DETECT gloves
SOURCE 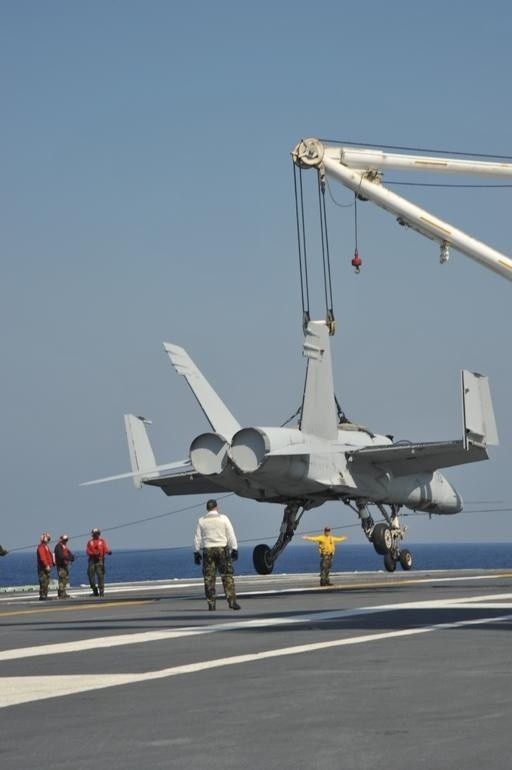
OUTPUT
[231,549,238,562]
[194,551,202,565]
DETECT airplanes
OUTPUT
[75,318,499,575]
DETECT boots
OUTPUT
[100,589,104,597]
[57,590,70,599]
[89,590,98,597]
[40,593,52,601]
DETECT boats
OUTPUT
[0,138,511,770]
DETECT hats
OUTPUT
[206,499,217,508]
[324,527,331,530]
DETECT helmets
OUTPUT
[59,534,69,543]
[90,528,101,538]
[41,532,51,543]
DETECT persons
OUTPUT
[54,534,74,599]
[193,500,241,610]
[303,527,347,587]
[37,533,54,599]
[86,528,112,597]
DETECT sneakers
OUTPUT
[208,605,215,611]
[229,599,241,610]
[319,581,334,586]
